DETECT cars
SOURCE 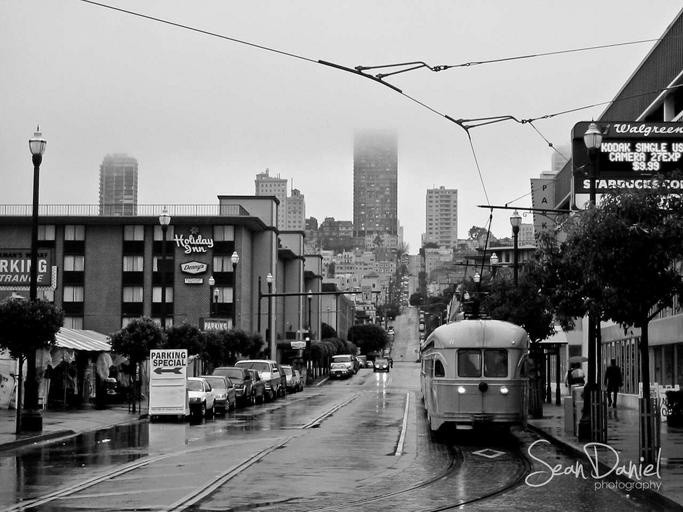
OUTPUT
[419,310,425,343]
[387,325,397,335]
[187,359,304,416]
[329,347,393,379]
[402,275,409,308]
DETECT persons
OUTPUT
[389,357,393,369]
[603,357,624,409]
[564,362,584,397]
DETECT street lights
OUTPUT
[307,290,313,337]
[578,119,602,443]
[266,272,273,358]
[159,204,171,330]
[208,276,220,318]
[462,251,499,315]
[20,122,48,432]
[510,208,522,280]
[231,250,239,330]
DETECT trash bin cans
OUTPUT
[665,390,683,428]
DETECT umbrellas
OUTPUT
[566,355,588,364]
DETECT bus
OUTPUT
[420,320,530,444]
